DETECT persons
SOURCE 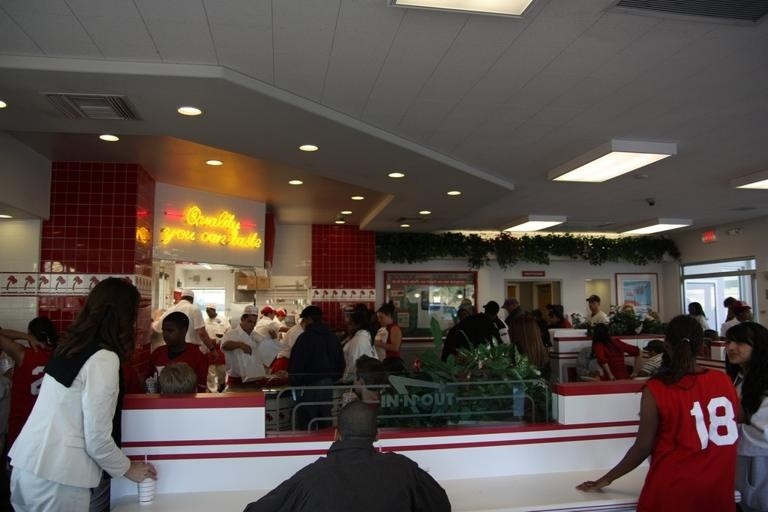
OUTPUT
[0,315,60,454]
[722,320,768,512]
[440,294,752,382]
[575,316,747,511]
[4,278,158,512]
[143,287,407,432]
[243,401,453,512]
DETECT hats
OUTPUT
[300,306,323,318]
[586,295,601,302]
[242,304,258,316]
[205,303,216,310]
[260,305,287,317]
[181,290,194,299]
[502,298,519,309]
[642,340,665,353]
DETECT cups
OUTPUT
[137,465,156,506]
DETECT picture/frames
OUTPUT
[615,273,660,314]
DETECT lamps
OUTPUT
[614,217,694,237]
[388,0,533,21]
[546,140,678,185]
[730,169,767,192]
[497,213,566,233]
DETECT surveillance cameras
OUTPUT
[648,199,655,205]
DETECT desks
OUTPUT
[111,463,652,511]
